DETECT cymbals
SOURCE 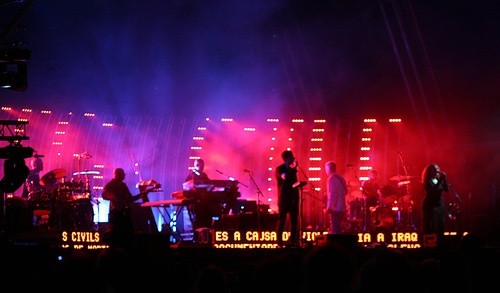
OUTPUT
[41,168,66,184]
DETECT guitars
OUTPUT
[110,183,161,210]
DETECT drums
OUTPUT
[378,185,396,205]
[348,201,364,222]
[370,204,397,231]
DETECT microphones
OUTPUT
[215,169,223,174]
[437,170,444,174]
[244,169,249,172]
[346,164,352,166]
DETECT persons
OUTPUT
[422,163,448,233]
[276,151,300,247]
[185,159,209,185]
[361,170,378,230]
[0,224,500,293]
[326,161,345,233]
[102,168,143,222]
[42,173,57,189]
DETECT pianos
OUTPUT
[140,198,192,242]
